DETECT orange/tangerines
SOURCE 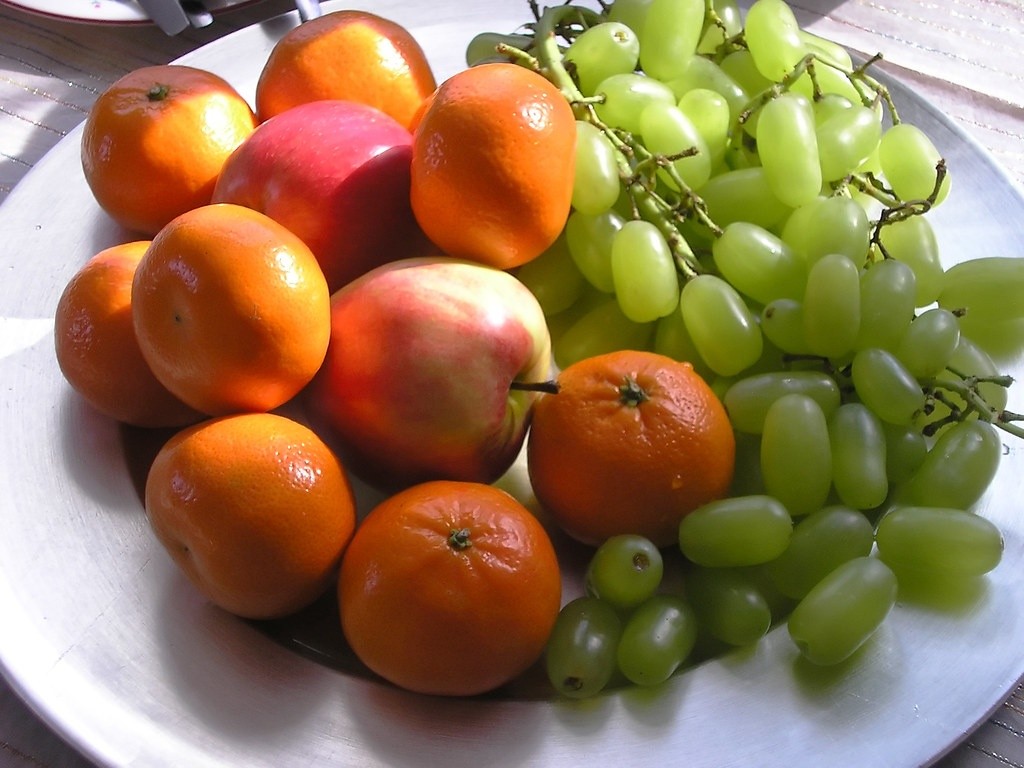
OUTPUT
[56,10,734,697]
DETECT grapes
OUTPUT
[466,0,1024,692]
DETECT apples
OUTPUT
[304,257,551,481]
[211,100,413,285]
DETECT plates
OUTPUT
[0,0,275,26]
[0,0,1024,768]
